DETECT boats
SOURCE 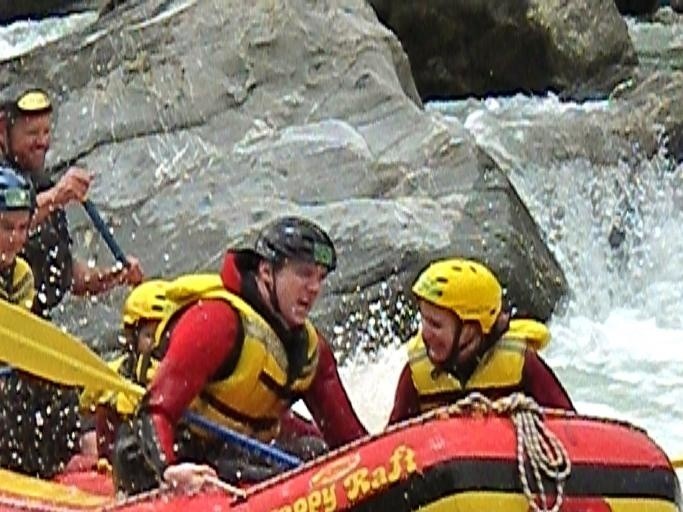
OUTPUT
[0,403,683,511]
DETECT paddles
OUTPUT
[0,300,305,467]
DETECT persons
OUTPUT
[380,256,578,419]
[0,164,83,480]
[0,79,146,329]
[86,276,178,471]
[114,212,372,490]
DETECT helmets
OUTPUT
[122,277,172,326]
[411,255,504,335]
[254,215,337,272]
[0,82,54,116]
[0,163,35,213]
